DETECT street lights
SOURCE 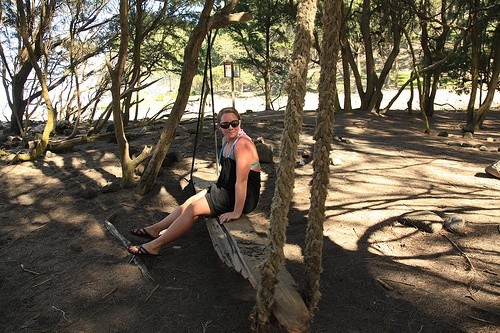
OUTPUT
[224,61,240,109]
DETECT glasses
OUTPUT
[219,119,241,129]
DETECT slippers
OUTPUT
[126,245,159,257]
[127,227,160,239]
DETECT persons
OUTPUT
[127,107,261,257]
